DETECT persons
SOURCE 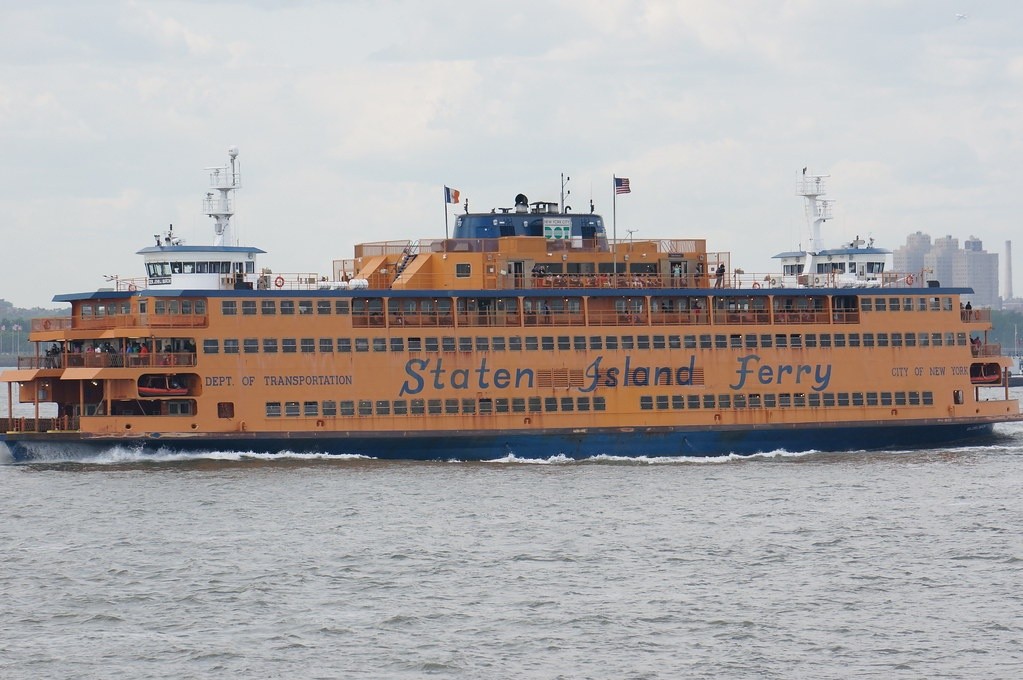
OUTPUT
[371,312,379,323]
[625,300,700,321]
[671,264,699,287]
[714,264,725,289]
[732,304,815,322]
[544,300,550,324]
[237,269,243,282]
[157,343,196,365]
[120,342,149,366]
[46,343,81,368]
[397,314,402,323]
[342,272,352,281]
[961,301,982,355]
[532,265,664,288]
[86,342,117,367]
[258,274,265,289]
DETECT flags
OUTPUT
[445,187,460,204]
[615,178,631,195]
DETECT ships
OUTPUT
[1,143,1022,460]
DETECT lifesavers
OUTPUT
[166,355,177,366]
[274,276,285,288]
[128,283,138,292]
[905,274,914,285]
[752,283,760,289]
[770,279,776,285]
[974,310,981,320]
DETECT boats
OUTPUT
[137,386,189,397]
[970,365,999,384]
